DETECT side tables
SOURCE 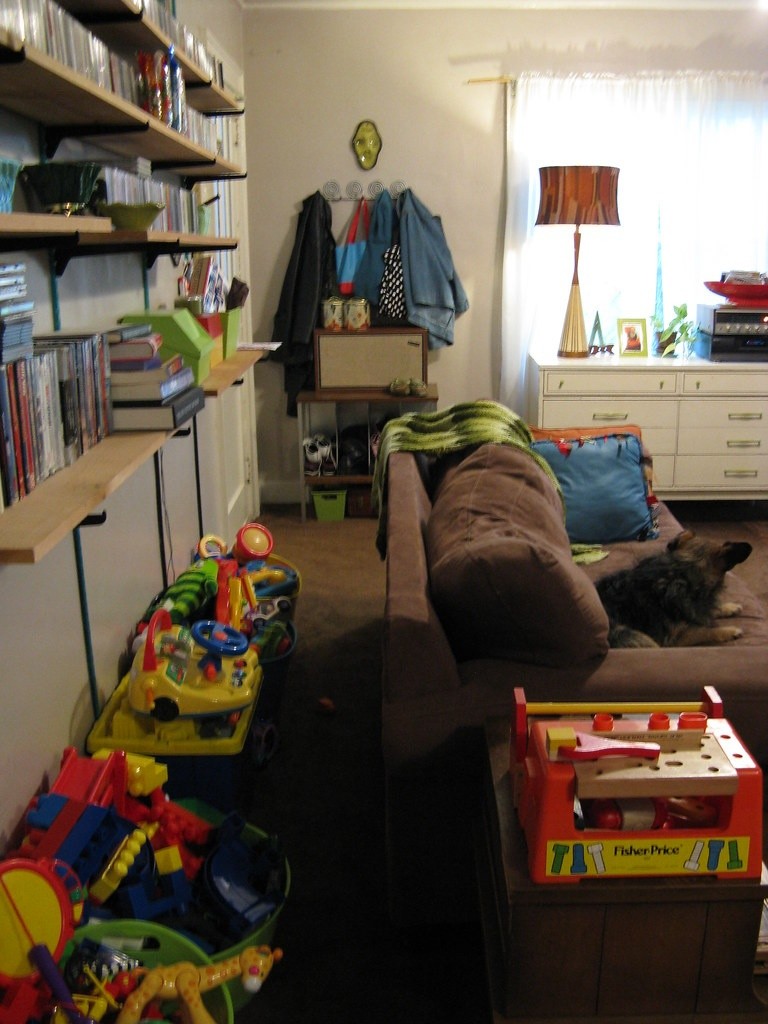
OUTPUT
[478,713,768,1024]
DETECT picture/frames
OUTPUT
[617,317,648,357]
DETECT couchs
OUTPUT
[372,398,768,926]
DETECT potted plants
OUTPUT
[650,304,696,359]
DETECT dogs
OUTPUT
[590,528,754,648]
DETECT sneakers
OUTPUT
[302,430,337,477]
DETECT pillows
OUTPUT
[426,443,609,667]
[533,432,655,544]
[528,425,642,443]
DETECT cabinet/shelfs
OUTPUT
[297,383,439,520]
[311,326,428,392]
[527,353,768,501]
[0,0,264,567]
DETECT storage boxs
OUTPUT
[257,551,302,620]
[59,918,235,1024]
[207,816,291,1008]
[309,486,348,522]
[218,306,242,360]
[257,613,297,683]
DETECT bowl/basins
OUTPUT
[703,281,768,308]
[17,160,167,233]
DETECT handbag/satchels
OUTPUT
[336,196,372,297]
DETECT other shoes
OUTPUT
[339,426,368,475]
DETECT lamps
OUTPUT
[535,166,620,359]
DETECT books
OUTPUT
[0,1,223,234]
[0,262,204,507]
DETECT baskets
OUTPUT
[348,491,377,518]
